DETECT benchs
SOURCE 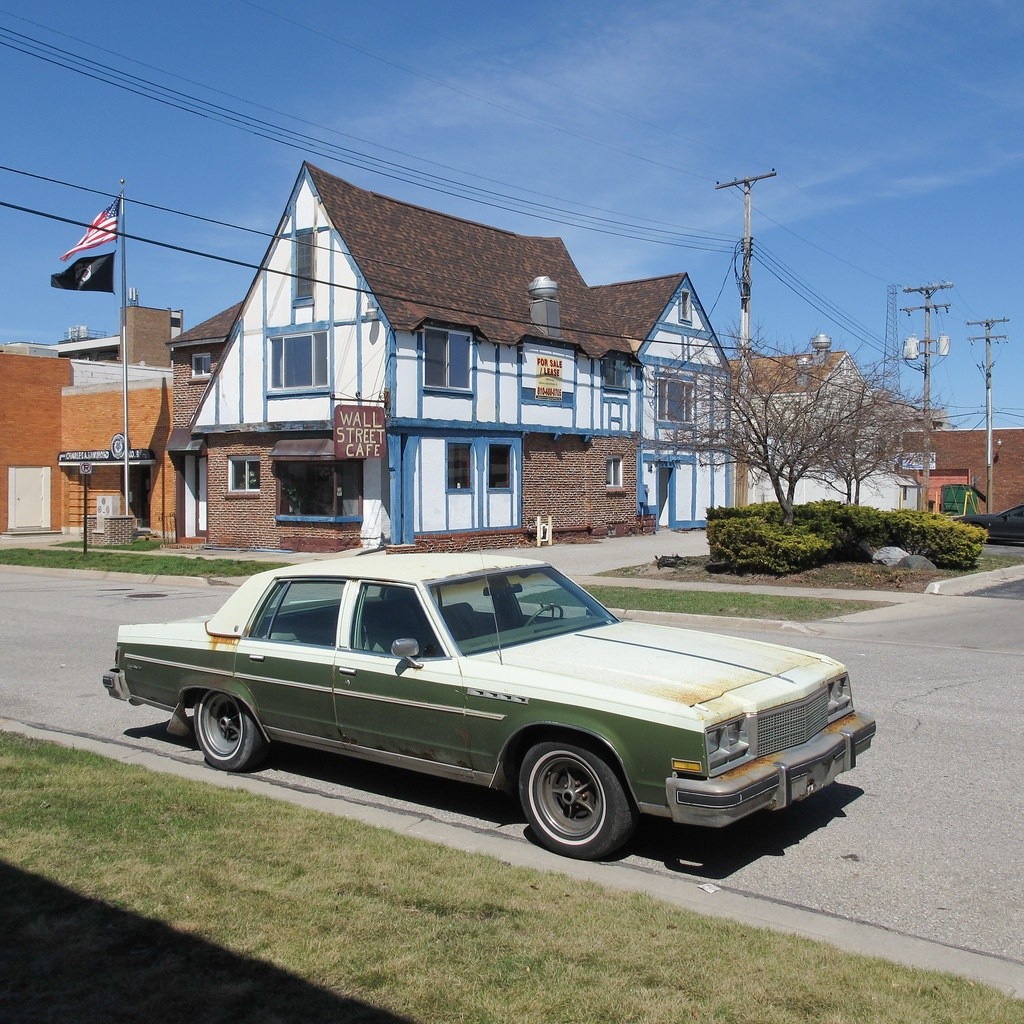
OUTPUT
[259,598,389,645]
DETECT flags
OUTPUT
[59,193,120,261]
[50,252,113,293]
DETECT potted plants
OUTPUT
[288,488,302,516]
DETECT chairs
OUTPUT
[366,602,431,656]
[437,600,480,641]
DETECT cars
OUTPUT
[953,502,1024,544]
[102,552,875,860]
